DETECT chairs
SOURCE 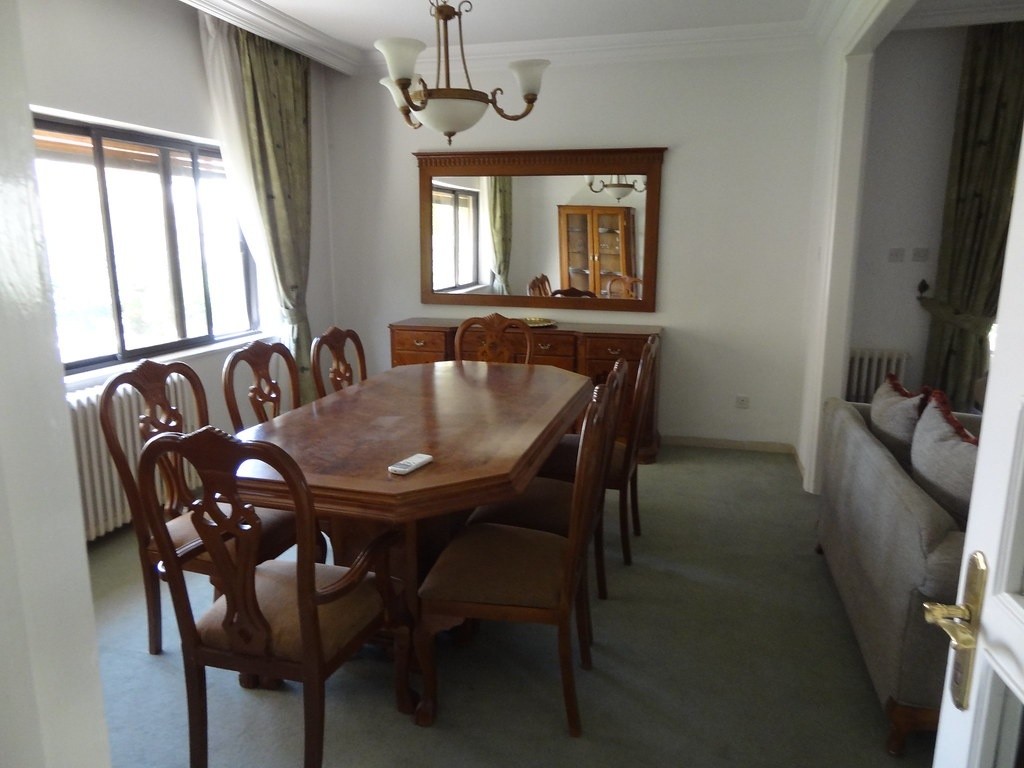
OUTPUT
[527,273,552,297]
[98,313,663,767]
[550,288,597,300]
[607,276,644,300]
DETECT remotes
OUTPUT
[389,453,433,475]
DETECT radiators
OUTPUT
[847,349,906,405]
[66,373,203,541]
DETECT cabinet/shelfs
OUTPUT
[387,317,664,464]
[556,204,638,299]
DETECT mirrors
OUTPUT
[413,147,669,313]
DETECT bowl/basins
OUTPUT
[599,227,609,233]
[569,267,583,273]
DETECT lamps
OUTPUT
[583,175,646,203]
[374,0,551,146]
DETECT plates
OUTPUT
[614,230,619,233]
[510,317,559,327]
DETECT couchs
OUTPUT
[813,397,982,754]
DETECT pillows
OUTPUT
[911,391,979,532]
[869,373,932,475]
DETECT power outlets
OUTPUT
[735,393,749,410]
[888,248,906,262]
[911,248,928,262]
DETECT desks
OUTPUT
[211,360,594,712]
